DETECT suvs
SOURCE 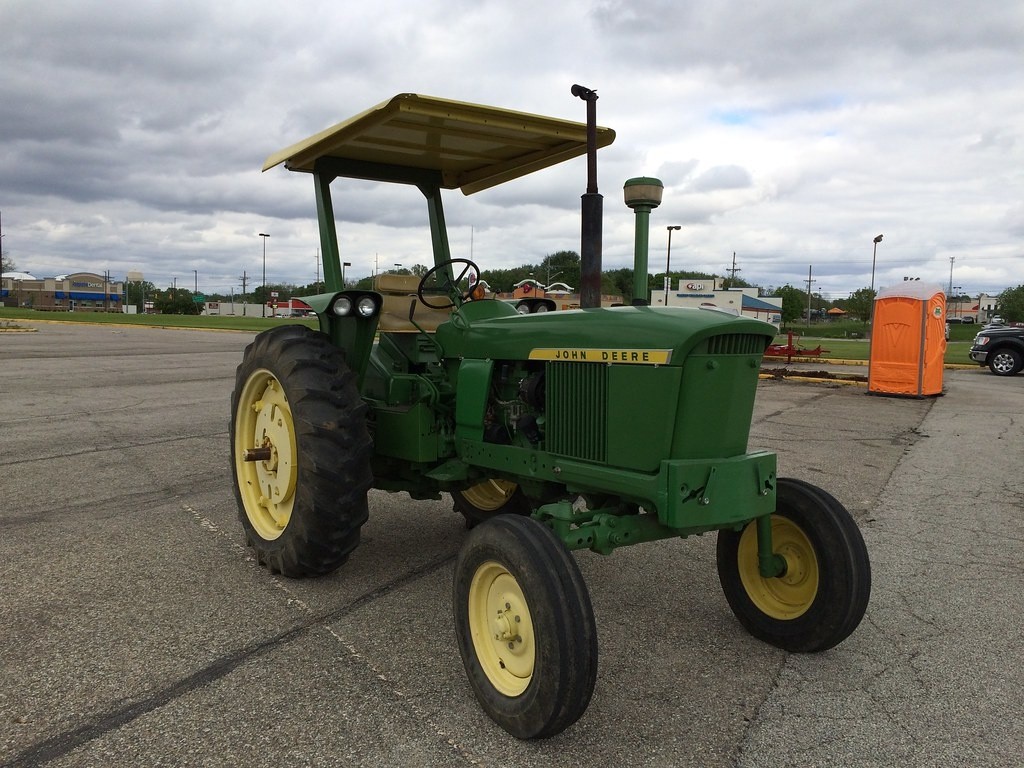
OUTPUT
[961,315,974,324]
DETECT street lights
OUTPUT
[953,287,961,317]
[959,293,966,316]
[395,264,402,274]
[665,226,681,305]
[871,234,883,324]
[342,262,351,286]
[547,271,563,286]
[258,233,270,318]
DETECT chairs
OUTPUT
[376,274,453,334]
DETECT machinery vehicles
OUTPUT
[228,83,871,741]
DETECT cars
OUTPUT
[982,323,1005,329]
[275,313,301,319]
[969,327,1024,375]
[992,316,1004,324]
